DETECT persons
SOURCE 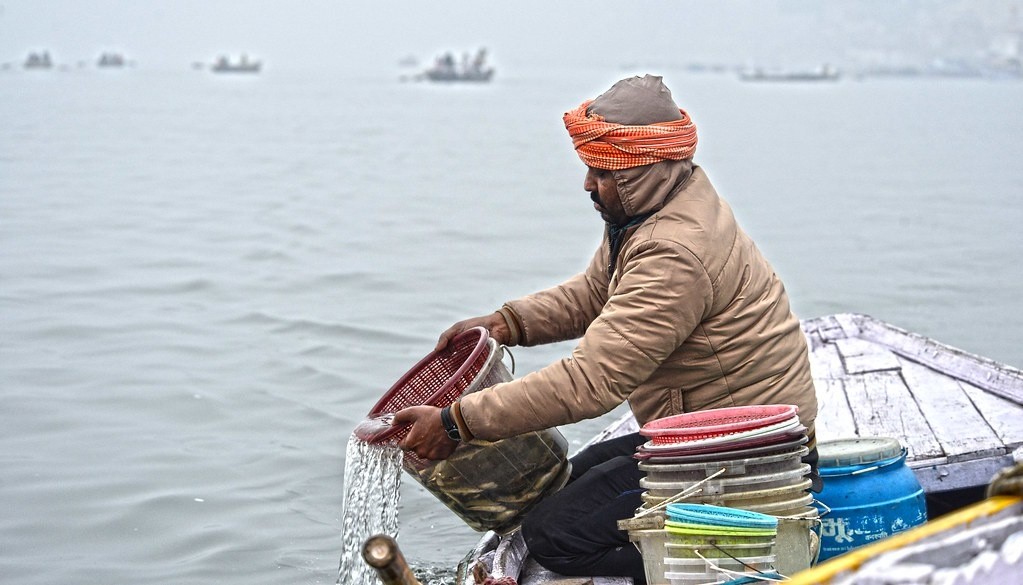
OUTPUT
[391,75,823,577]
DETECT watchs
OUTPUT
[440,406,462,442]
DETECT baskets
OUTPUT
[633,403,831,585]
[357,325,504,471]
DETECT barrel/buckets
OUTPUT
[354,336,573,537]
[812,434,927,563]
[616,404,830,584]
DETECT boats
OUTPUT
[427,69,494,81]
[740,71,842,79]
[212,60,261,72]
